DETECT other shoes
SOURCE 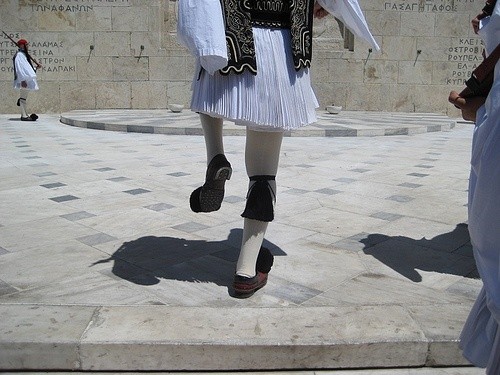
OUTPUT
[190,154,232,212]
[21,114,38,121]
[233,247,272,298]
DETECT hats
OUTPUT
[18,40,27,45]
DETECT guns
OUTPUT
[2,30,42,68]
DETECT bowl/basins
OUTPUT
[169,104,184,112]
[326,106,342,114]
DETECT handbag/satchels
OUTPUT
[455,44,500,121]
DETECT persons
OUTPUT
[12,39,41,121]
[449,1,500,375]
[178,0,379,298]
